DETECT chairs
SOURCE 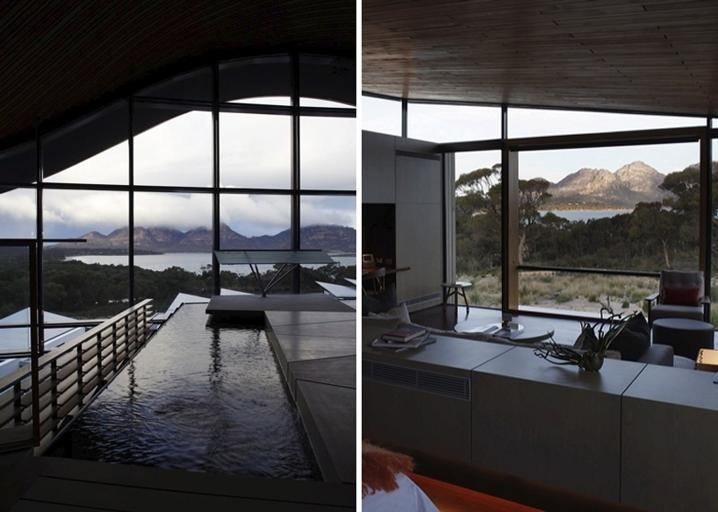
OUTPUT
[646,270,712,327]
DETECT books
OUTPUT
[380,322,427,342]
[374,336,438,354]
[370,332,430,350]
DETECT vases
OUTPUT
[584,351,604,372]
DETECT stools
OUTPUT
[652,318,715,361]
[440,281,472,314]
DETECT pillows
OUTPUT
[366,301,412,323]
